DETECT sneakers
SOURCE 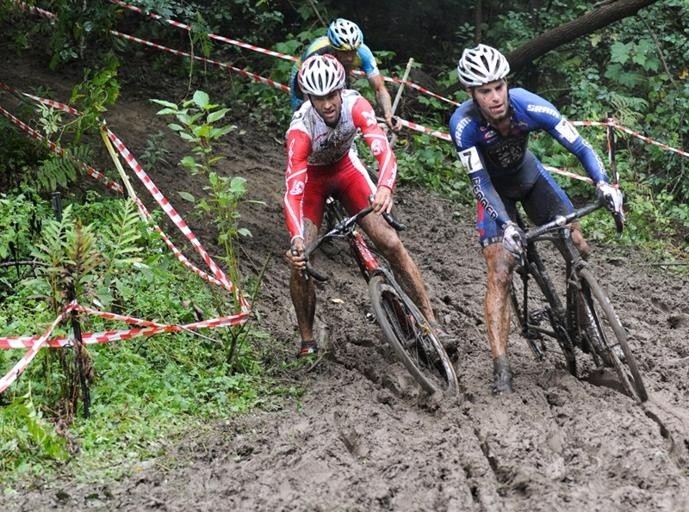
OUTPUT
[432,325,459,357]
[298,338,318,365]
[595,344,627,365]
[492,367,514,400]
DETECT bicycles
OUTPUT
[291,193,460,402]
[502,185,648,403]
[354,115,402,240]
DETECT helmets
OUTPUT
[457,43,511,89]
[297,52,346,98]
[327,18,364,51]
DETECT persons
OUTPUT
[449,41,629,407]
[280,55,463,374]
[286,16,405,138]
